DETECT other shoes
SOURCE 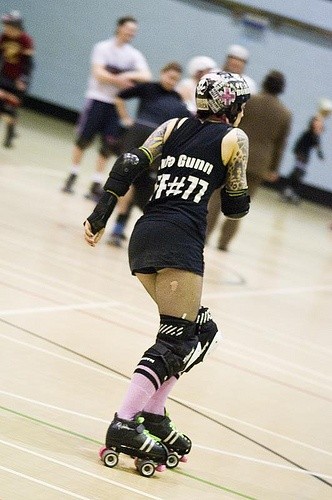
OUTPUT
[4,139,14,147]
[89,183,103,197]
[63,184,73,194]
[85,192,104,202]
[109,232,128,247]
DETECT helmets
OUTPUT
[189,55,216,77]
[194,70,252,122]
[1,8,23,26]
[227,44,250,63]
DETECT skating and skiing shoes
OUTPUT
[99,411,169,476]
[135,409,192,468]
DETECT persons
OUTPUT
[0,9,35,150]
[60,16,332,252]
[82,70,252,476]
